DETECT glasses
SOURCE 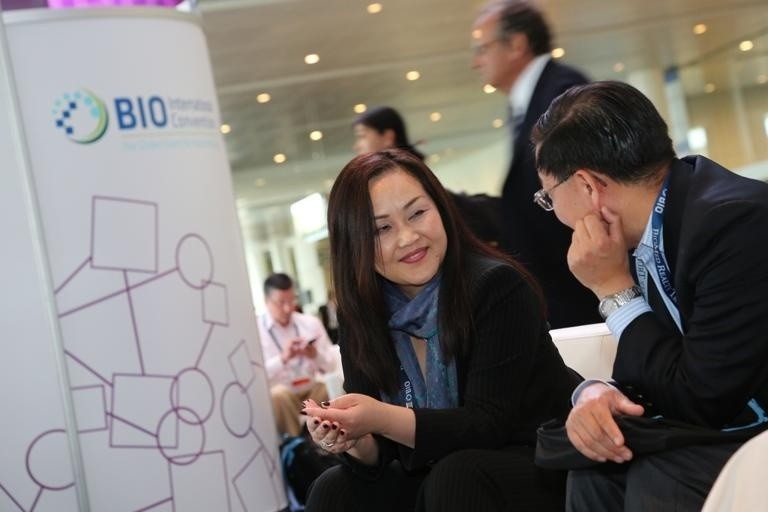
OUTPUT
[534,169,607,213]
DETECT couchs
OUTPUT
[539,319,767,510]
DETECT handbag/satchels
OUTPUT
[530,411,700,468]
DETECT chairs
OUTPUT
[314,343,348,403]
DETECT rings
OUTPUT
[322,441,333,447]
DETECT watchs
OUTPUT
[598,286,644,320]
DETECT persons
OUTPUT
[350,106,426,161]
[527,81,768,511]
[258,272,337,440]
[299,148,585,512]
[470,0,604,329]
[318,289,341,344]
